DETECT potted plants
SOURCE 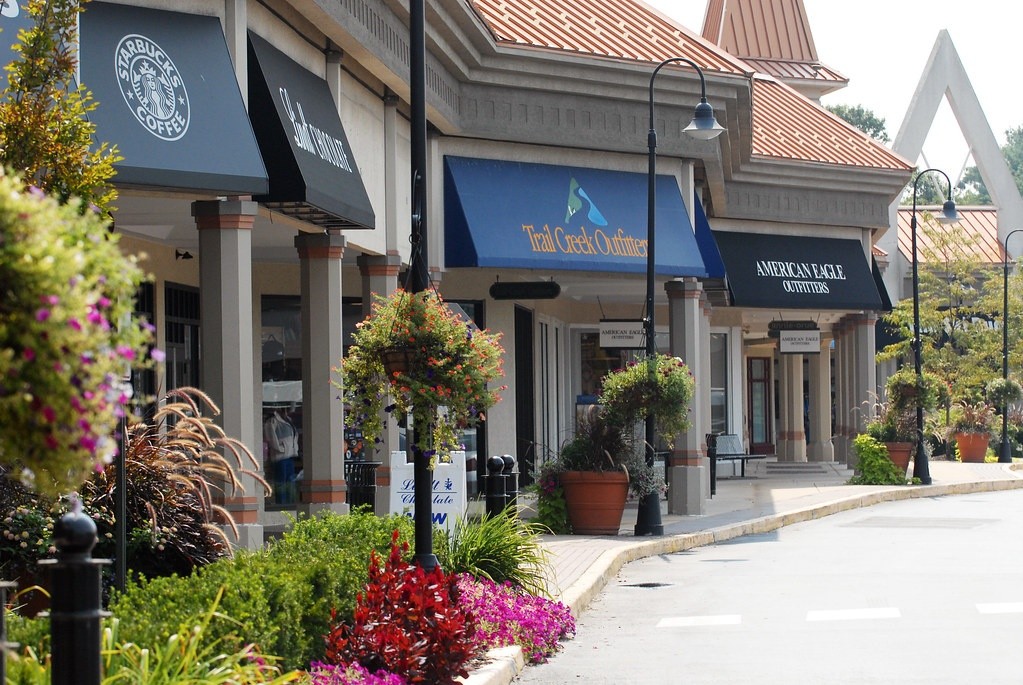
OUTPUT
[955,418,991,464]
[847,405,925,486]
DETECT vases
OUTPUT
[377,345,424,388]
[900,385,919,396]
[564,469,632,535]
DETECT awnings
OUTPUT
[0,0,99,159]
[876,305,1001,353]
[247,28,376,229]
[444,155,707,276]
[694,189,726,278]
[712,230,882,310]
[78,0,270,194]
[872,254,892,310]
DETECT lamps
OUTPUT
[174,248,193,261]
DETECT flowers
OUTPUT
[985,376,1023,405]
[523,351,696,535]
[886,363,952,402]
[339,284,508,469]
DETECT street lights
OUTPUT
[912,167,966,486]
[635,56,730,535]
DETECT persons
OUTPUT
[264,401,297,505]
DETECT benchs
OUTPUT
[716,434,767,477]
